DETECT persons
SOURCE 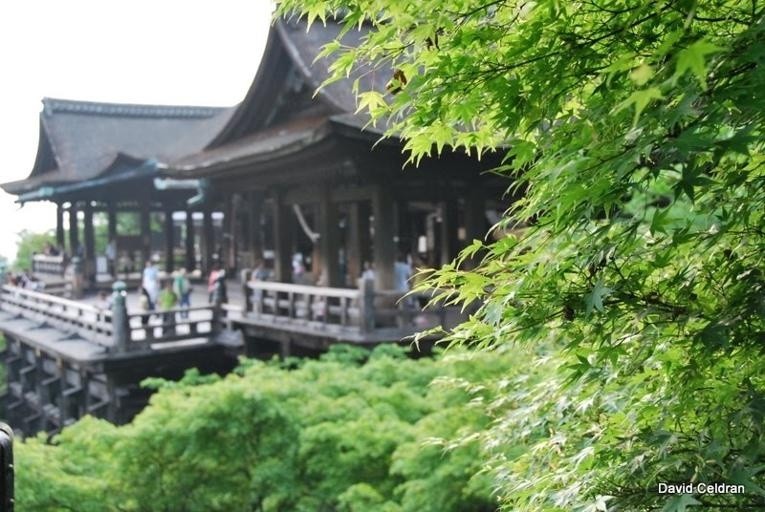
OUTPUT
[103,234,134,279]
[206,259,226,305]
[7,268,39,291]
[290,248,306,274]
[392,254,431,308]
[42,243,60,256]
[338,242,345,282]
[362,260,377,278]
[252,259,269,299]
[136,260,194,319]
